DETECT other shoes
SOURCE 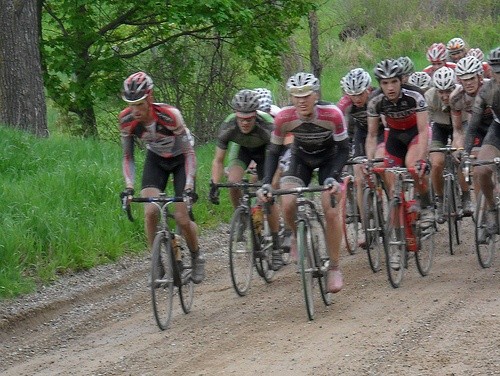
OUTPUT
[271,248,284,270]
[462,199,474,215]
[484,210,498,235]
[420,204,435,228]
[191,252,206,283]
[357,229,366,245]
[390,248,402,269]
[436,203,445,224]
[325,269,344,293]
[148,264,165,287]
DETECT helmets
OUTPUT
[339,67,372,96]
[485,46,500,72]
[373,57,405,80]
[408,71,432,90]
[398,56,415,75]
[231,88,261,119]
[455,56,483,80]
[121,71,155,104]
[433,66,456,90]
[464,48,484,62]
[253,88,273,111]
[285,71,321,97]
[446,37,466,55]
[426,42,449,62]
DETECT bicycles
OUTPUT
[429,146,500,266]
[260,181,334,318]
[335,156,437,286]
[211,178,290,298]
[121,194,193,330]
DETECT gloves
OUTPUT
[209,186,221,205]
[413,159,431,178]
[359,160,373,176]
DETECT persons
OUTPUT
[210,37,500,292]
[117,72,204,284]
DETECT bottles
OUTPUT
[252,204,264,233]
[169,232,183,261]
